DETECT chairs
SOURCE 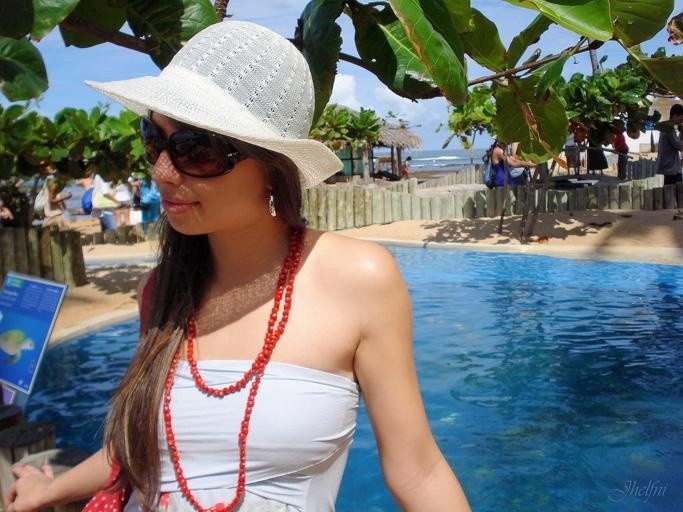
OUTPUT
[1,173,160,246]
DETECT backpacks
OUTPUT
[482,157,496,190]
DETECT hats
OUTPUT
[80,19,346,191]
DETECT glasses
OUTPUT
[138,116,258,179]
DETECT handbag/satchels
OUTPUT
[82,184,130,213]
[132,191,150,211]
[33,187,65,218]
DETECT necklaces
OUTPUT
[160,222,306,512]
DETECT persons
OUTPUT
[480,102,683,192]
[7,19,476,512]
[403,157,411,178]
[33,167,162,240]
